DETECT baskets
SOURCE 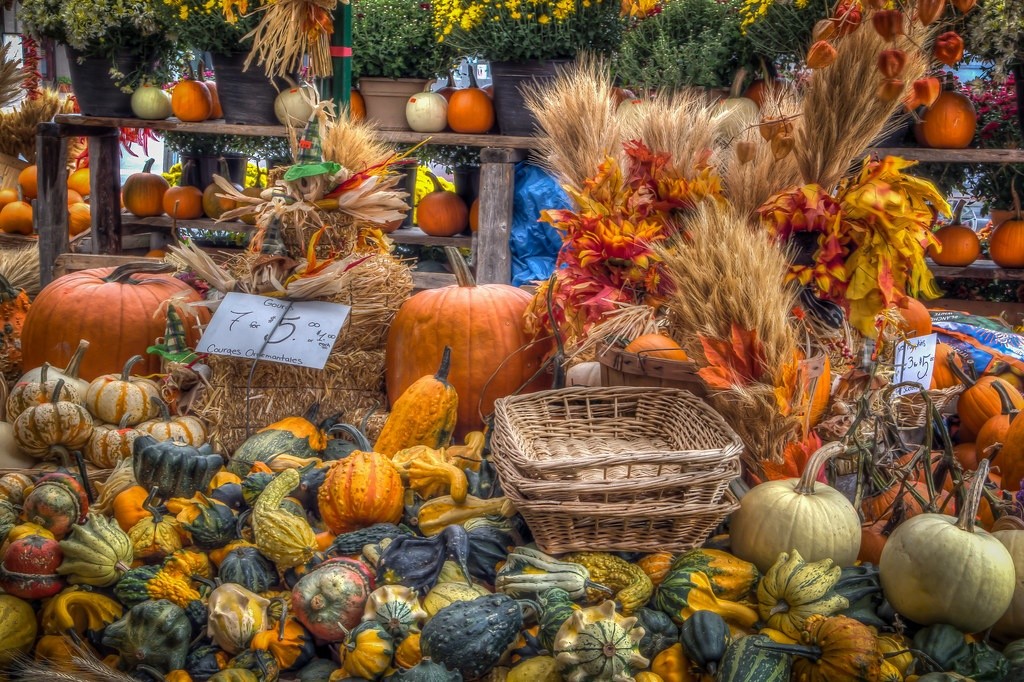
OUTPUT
[489,387,746,556]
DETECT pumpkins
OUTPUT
[0,69,1024,682]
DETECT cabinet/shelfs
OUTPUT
[34,113,1024,298]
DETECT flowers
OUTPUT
[519,140,950,369]
[17,0,1023,90]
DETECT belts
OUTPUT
[895,383,966,429]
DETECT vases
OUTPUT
[662,85,730,106]
[357,76,428,131]
[64,45,137,119]
[208,50,298,126]
[489,58,575,137]
[1010,64,1024,137]
[862,80,913,150]
